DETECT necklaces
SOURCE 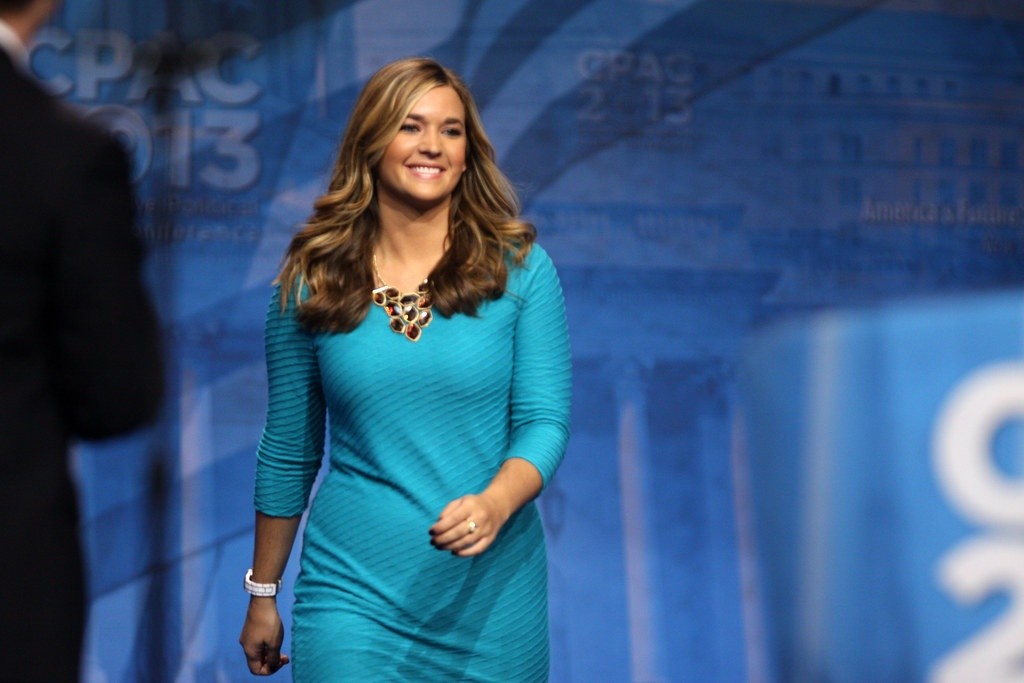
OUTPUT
[371,247,435,343]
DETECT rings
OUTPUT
[468,519,476,532]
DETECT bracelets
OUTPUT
[242,568,284,598]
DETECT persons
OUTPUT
[0,0,169,683]
[238,55,575,683]
